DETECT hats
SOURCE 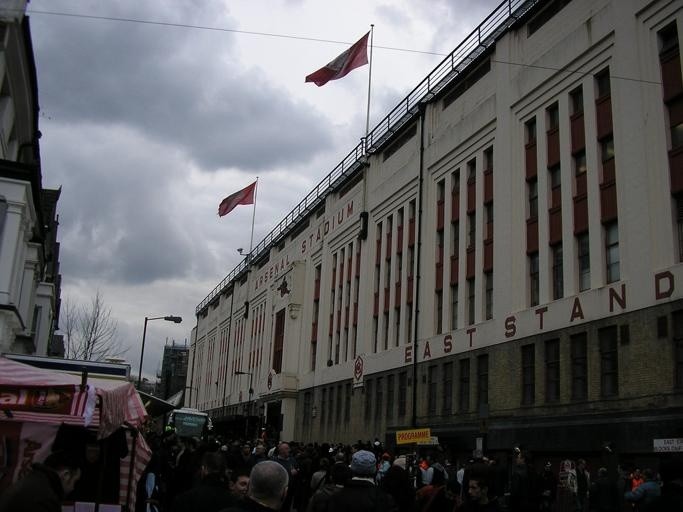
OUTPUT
[349,449,378,476]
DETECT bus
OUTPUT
[164,406,213,440]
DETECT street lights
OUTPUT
[234,371,252,442]
[138,316,182,392]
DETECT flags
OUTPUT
[217,180,256,217]
[305,28,371,87]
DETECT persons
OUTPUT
[0,445,80,512]
